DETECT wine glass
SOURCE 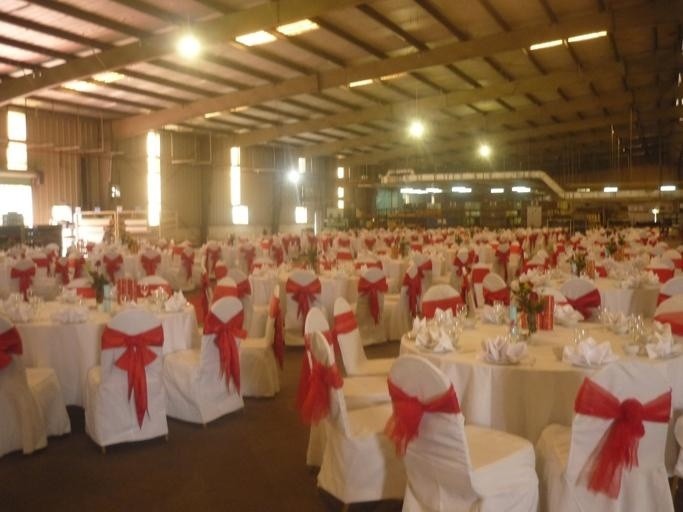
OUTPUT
[595,301,646,337]
[434,312,443,324]
[504,327,514,343]
[449,319,462,328]
[119,294,129,306]
[29,297,40,308]
[76,297,86,306]
[140,282,149,296]
[574,328,584,343]
[494,300,503,312]
[11,295,22,311]
[456,304,467,319]
[26,289,32,299]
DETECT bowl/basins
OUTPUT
[623,344,638,355]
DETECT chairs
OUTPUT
[499,226,683,337]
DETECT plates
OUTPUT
[160,310,184,313]
[640,353,681,359]
[574,361,611,368]
[416,345,460,352]
[60,321,87,324]
[12,320,33,323]
[489,361,518,365]
